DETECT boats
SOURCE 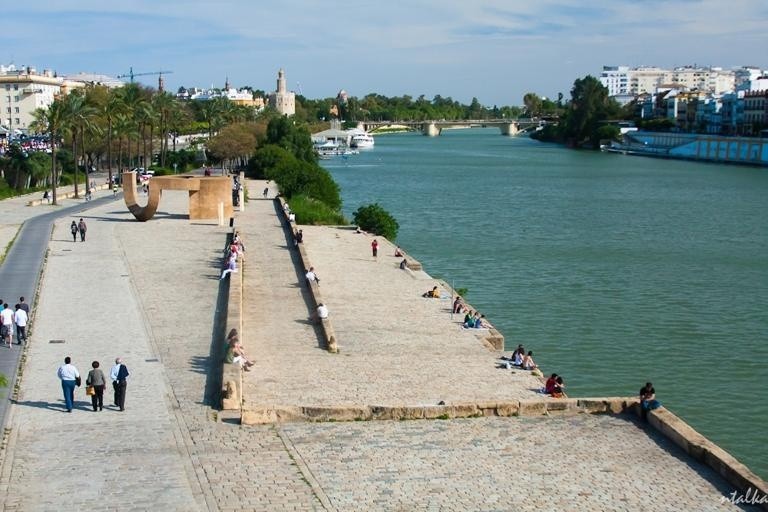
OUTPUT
[313,138,358,157]
[351,133,375,147]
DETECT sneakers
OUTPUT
[120,410,125,412]
[245,364,254,373]
[94,408,103,411]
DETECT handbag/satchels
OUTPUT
[86,386,96,395]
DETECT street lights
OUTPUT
[541,96,546,115]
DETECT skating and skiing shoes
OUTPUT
[67,410,72,413]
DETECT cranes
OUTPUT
[117,67,173,83]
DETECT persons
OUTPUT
[110,358,129,411]
[545,373,566,394]
[453,296,492,329]
[356,227,366,234]
[225,328,256,371]
[219,231,246,282]
[430,286,441,299]
[395,245,404,257]
[79,218,88,241]
[283,199,303,247]
[43,189,51,202]
[84,174,118,203]
[304,265,327,324]
[0,296,32,349]
[400,259,412,273]
[372,239,379,257]
[70,220,78,242]
[510,343,537,369]
[57,357,81,413]
[86,361,107,412]
[640,381,661,420]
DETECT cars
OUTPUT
[112,167,154,185]
